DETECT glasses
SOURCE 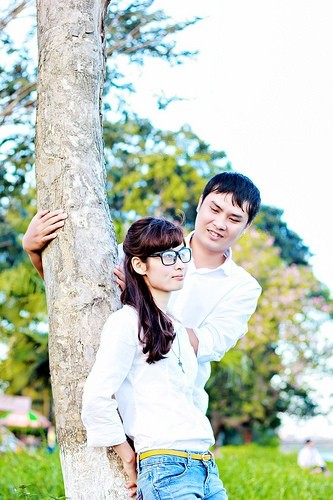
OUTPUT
[147,246,192,267]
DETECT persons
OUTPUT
[20,172,265,416]
[80,216,230,499]
[294,437,329,474]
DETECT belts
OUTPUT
[136,450,215,462]
